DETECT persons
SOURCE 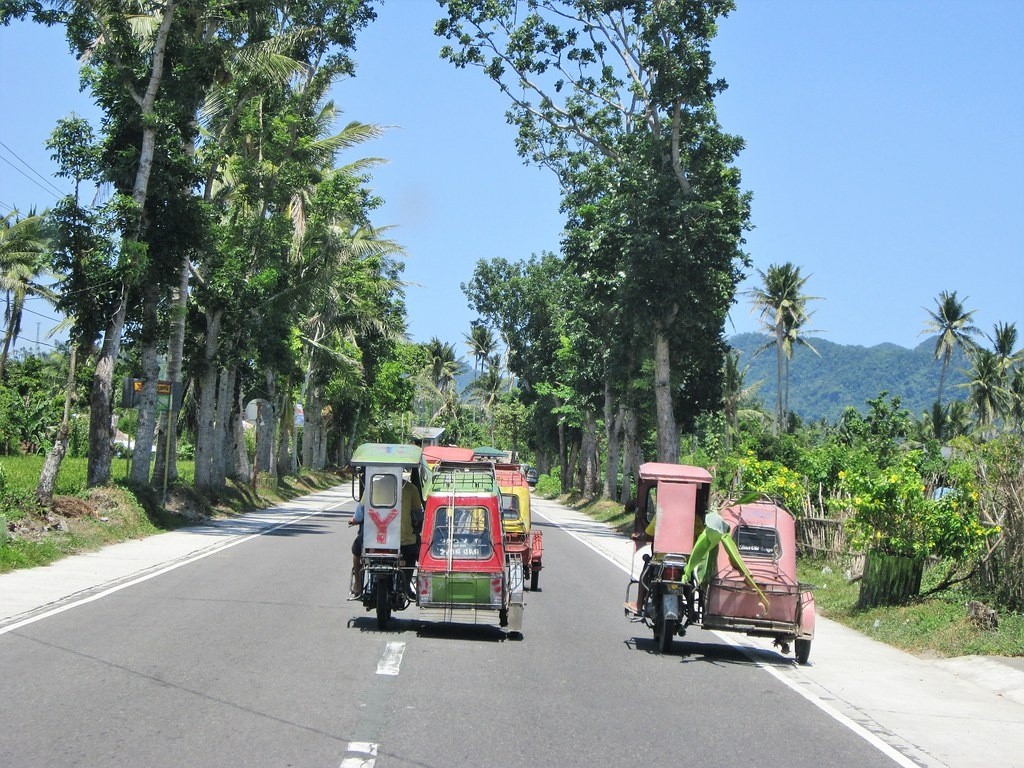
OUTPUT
[623,491,705,623]
[348,471,424,605]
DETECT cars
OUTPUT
[526,468,538,484]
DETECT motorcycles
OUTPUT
[352,441,425,626]
[624,461,714,654]
